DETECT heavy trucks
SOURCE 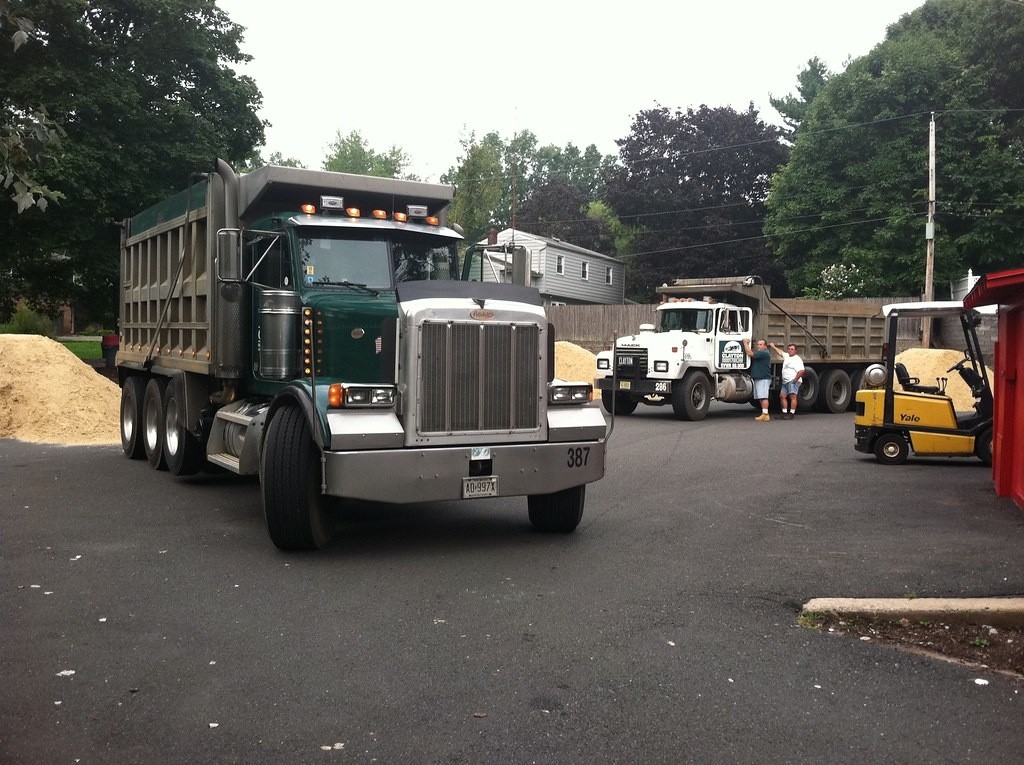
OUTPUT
[116,156,617,553]
[594,274,887,422]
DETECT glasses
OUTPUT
[788,348,795,350]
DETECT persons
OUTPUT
[743,338,772,420]
[770,342,805,420]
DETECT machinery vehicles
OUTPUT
[855,299,994,464]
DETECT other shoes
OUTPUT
[755,413,770,420]
[783,413,793,420]
[774,412,788,419]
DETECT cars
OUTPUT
[101,317,120,372]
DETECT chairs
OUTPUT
[896,363,938,396]
